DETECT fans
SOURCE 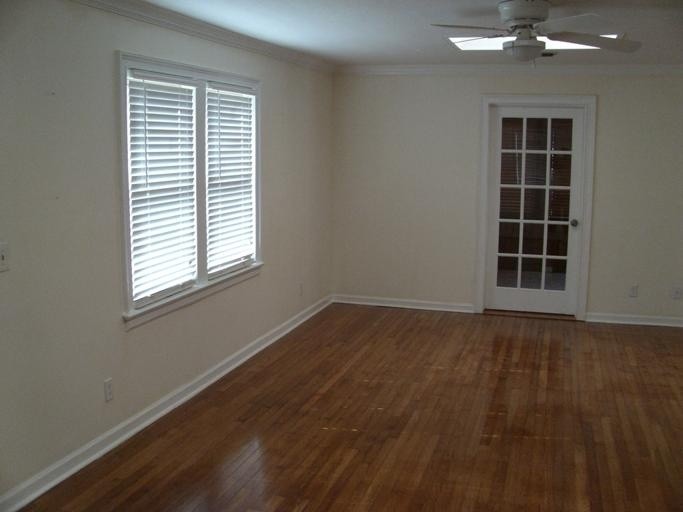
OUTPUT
[430,0,642,62]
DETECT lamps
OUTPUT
[501,31,547,65]
